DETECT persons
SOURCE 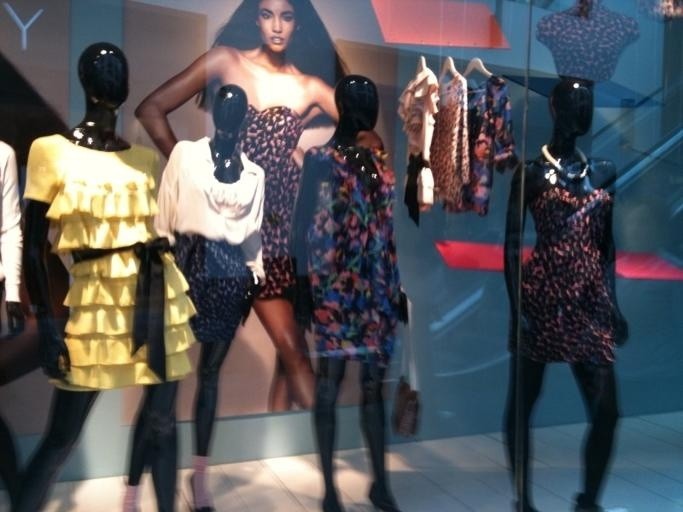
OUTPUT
[501,80,629,512]
[8,41,198,512]
[0,137,28,337]
[123,85,266,512]
[288,73,409,512]
[134,0,346,415]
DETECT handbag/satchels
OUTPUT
[393,298,419,434]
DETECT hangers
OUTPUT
[439,56,466,84]
[462,58,495,84]
[403,55,438,81]
